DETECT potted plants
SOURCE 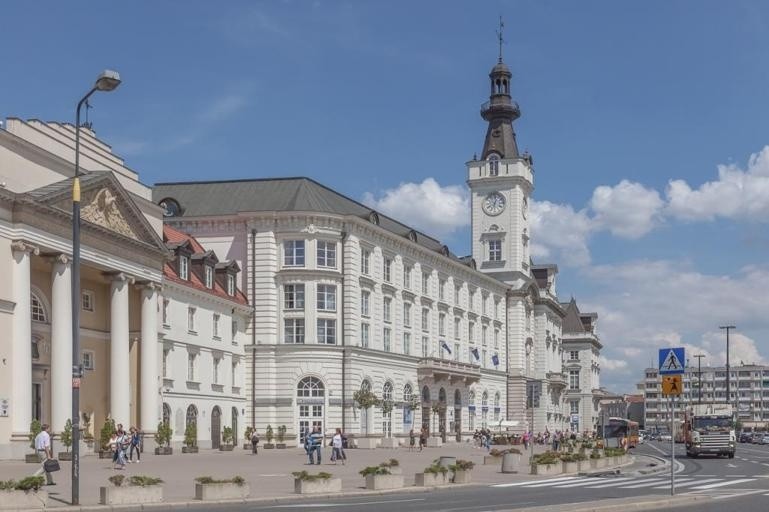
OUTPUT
[413,460,451,487]
[182,420,199,453]
[98,474,166,506]
[219,425,236,452]
[359,460,404,492]
[263,424,275,450]
[193,475,251,502]
[1,473,50,510]
[291,469,342,495]
[277,424,287,450]
[560,437,630,473]
[154,421,175,456]
[25,421,42,464]
[528,450,562,477]
[499,448,522,474]
[243,425,257,451]
[453,458,475,485]
[58,418,73,462]
[99,421,119,459]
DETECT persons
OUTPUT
[330,428,349,465]
[303,427,311,455]
[305,425,324,464]
[249,428,258,455]
[104,423,141,471]
[492,355,499,365]
[472,349,479,360]
[442,343,451,354]
[32,423,57,485]
[408,425,596,453]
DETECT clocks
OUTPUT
[481,189,507,216]
[522,196,529,220]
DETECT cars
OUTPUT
[649,433,659,441]
[734,429,769,445]
[659,432,672,441]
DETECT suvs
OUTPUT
[638,434,643,444]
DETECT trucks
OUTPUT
[684,403,736,459]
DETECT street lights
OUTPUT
[692,353,708,403]
[718,325,740,404]
[65,56,125,506]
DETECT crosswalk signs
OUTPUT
[656,346,687,374]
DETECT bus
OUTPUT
[638,430,647,439]
[673,420,685,442]
[595,418,639,452]
